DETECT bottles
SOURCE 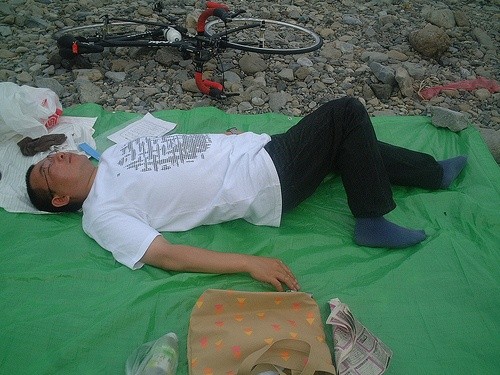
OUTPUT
[143,332,179,375]
[162,27,182,41]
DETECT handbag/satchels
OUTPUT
[185,287,338,374]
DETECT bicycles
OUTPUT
[52,1,324,104]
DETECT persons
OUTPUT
[26,96,468,293]
